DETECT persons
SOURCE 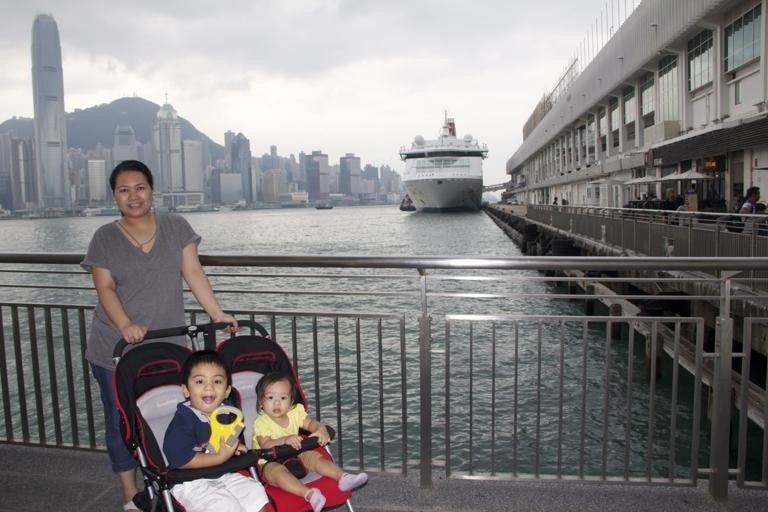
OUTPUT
[76,158,241,512]
[560,199,567,212]
[624,184,767,236]
[552,196,559,211]
[249,367,369,512]
[162,347,272,512]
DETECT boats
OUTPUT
[398,109,489,214]
[315,202,333,209]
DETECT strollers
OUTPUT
[112,319,355,512]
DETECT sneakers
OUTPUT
[339,472,368,491]
[304,487,326,512]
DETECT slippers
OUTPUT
[122,501,141,511]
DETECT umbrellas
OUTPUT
[633,175,661,197]
[655,170,684,195]
[622,174,646,200]
[668,169,713,193]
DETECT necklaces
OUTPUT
[116,220,156,254]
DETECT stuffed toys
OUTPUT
[200,403,246,457]
